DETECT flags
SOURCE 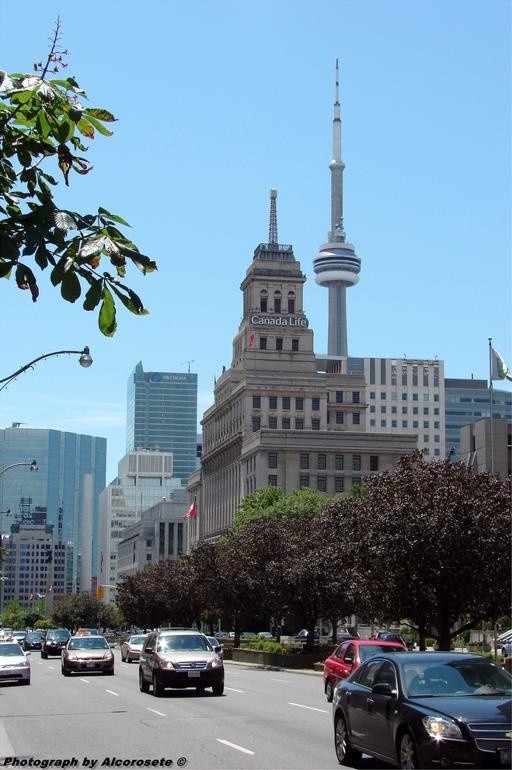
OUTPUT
[177,502,198,520]
[490,345,512,381]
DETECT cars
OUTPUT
[0,628,273,696]
[295,629,319,643]
[323,631,512,770]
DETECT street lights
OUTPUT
[0,510,12,516]
[0,345,93,390]
[0,459,38,474]
